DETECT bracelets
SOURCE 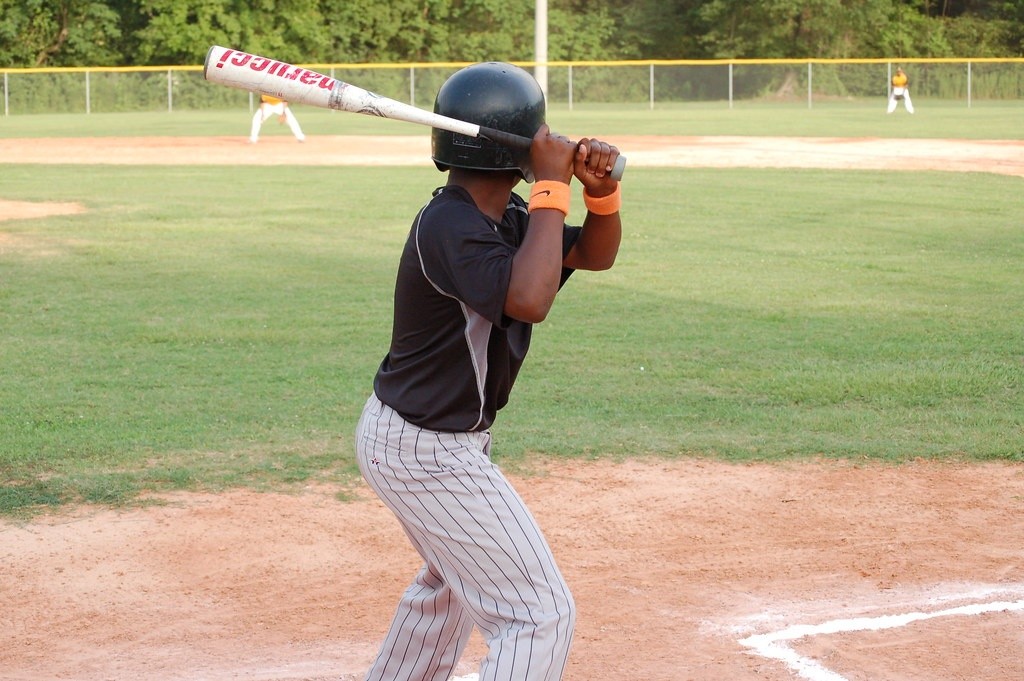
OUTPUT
[582,181,621,215]
[528,181,570,216]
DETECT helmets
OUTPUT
[431,62,545,184]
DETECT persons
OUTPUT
[352,62,621,681]
[887,66,914,114]
[251,94,306,142]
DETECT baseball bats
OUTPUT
[201,44,628,182]
